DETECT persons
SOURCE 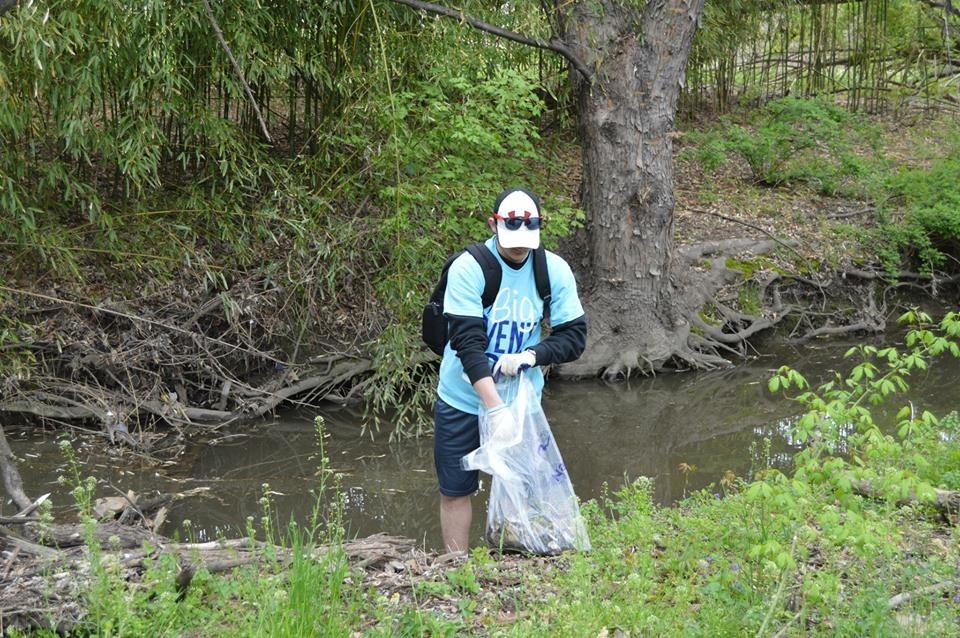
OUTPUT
[422,189,588,564]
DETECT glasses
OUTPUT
[492,211,545,230]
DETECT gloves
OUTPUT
[487,403,516,443]
[490,349,536,381]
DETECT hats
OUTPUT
[492,187,542,250]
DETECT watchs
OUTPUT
[526,348,536,359]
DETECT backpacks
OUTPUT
[420,243,552,358]
